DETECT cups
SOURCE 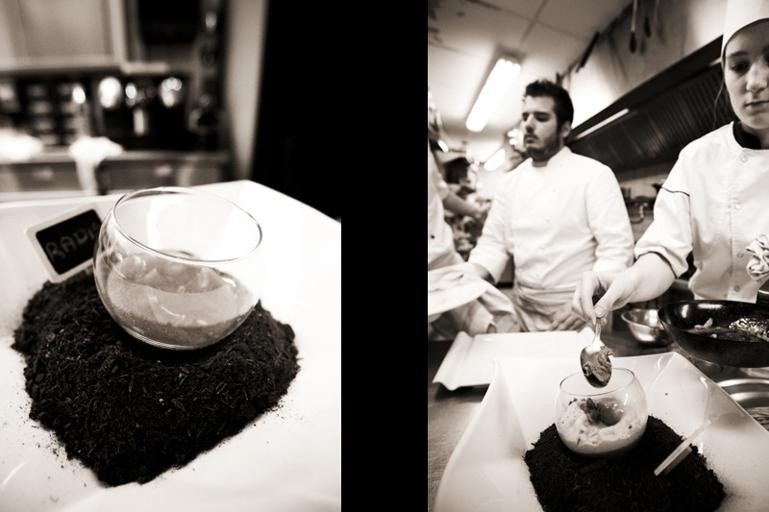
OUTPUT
[94,189,268,350]
[556,368,601,452]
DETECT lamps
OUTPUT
[464,56,521,133]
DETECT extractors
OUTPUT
[563,49,744,183]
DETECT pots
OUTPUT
[658,299,769,369]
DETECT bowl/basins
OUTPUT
[621,308,672,345]
[714,379,769,428]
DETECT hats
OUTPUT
[721,0,769,69]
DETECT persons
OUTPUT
[571,0,769,333]
[428,111,490,264]
[428,151,514,343]
[467,80,637,333]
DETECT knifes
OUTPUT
[655,424,703,477]
